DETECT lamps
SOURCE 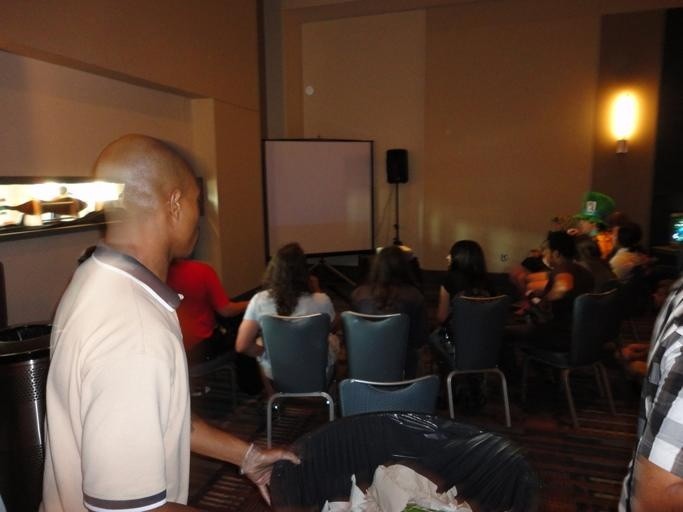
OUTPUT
[608,95,637,162]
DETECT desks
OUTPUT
[648,245,683,277]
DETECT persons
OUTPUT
[503,189,669,415]
[36,132,303,512]
[426,240,497,417]
[166,254,264,398]
[232,247,342,407]
[349,246,430,379]
[616,273,683,512]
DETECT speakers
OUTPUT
[386,149,409,183]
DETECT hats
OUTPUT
[572,191,616,228]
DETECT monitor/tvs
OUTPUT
[670,214,683,246]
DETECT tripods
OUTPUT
[302,258,357,288]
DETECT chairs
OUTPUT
[429,294,518,426]
[189,349,238,408]
[259,312,334,449]
[336,310,442,419]
[518,288,617,437]
[594,258,660,359]
